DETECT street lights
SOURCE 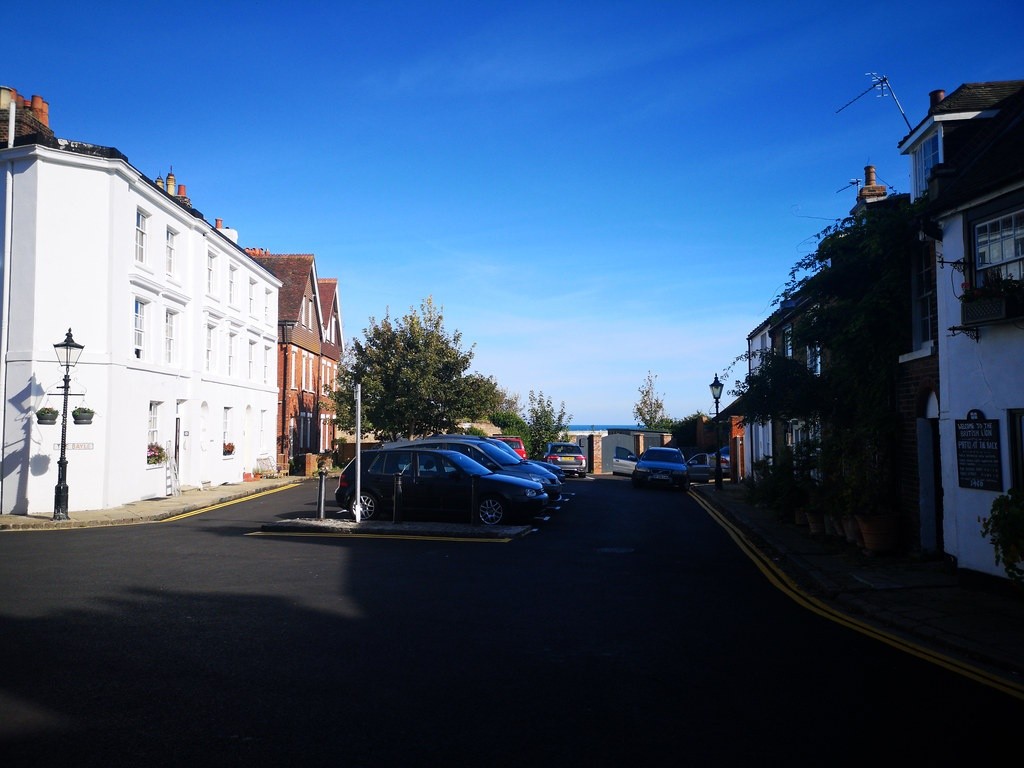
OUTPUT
[709,374,725,492]
[53,327,81,522]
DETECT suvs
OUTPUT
[428,435,568,486]
[367,439,562,510]
[613,446,710,490]
[492,436,528,461]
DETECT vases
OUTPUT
[742,199,931,551]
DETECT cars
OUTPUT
[706,446,730,477]
[544,443,587,478]
[335,448,548,524]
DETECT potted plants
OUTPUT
[71,406,95,424]
[35,407,59,425]
